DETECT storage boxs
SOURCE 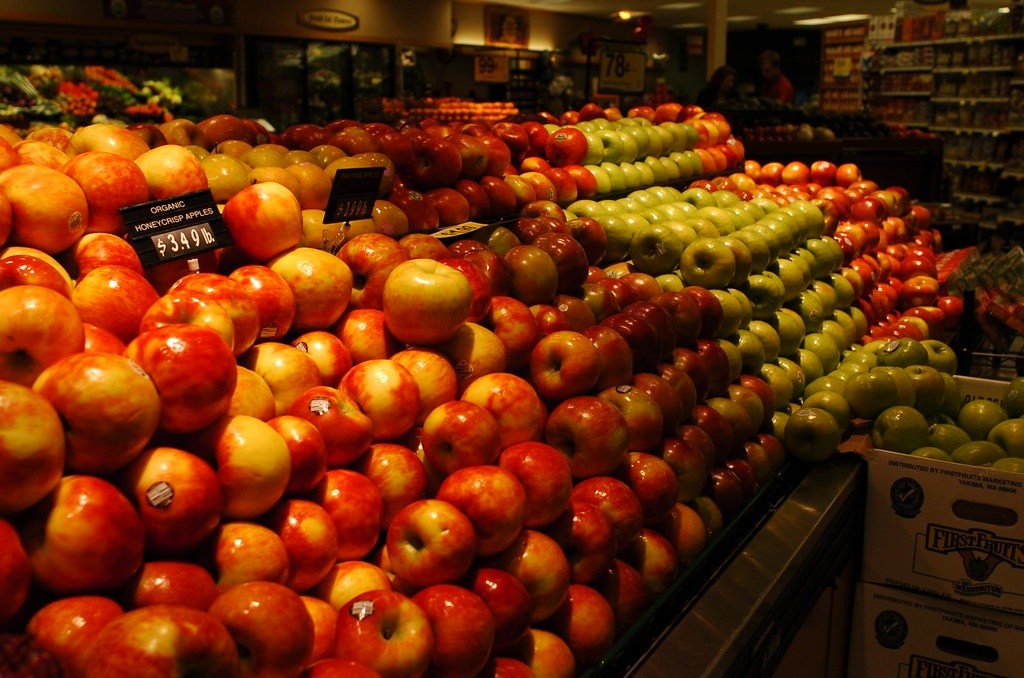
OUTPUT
[837,376,1024,616]
[850,577,1024,678]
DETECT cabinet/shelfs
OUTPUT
[243,33,553,128]
[865,33,1023,239]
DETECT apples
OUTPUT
[0,99,1024,678]
[57,66,172,118]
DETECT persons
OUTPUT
[547,75,577,118]
[641,51,793,116]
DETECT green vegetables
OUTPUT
[0,65,149,118]
